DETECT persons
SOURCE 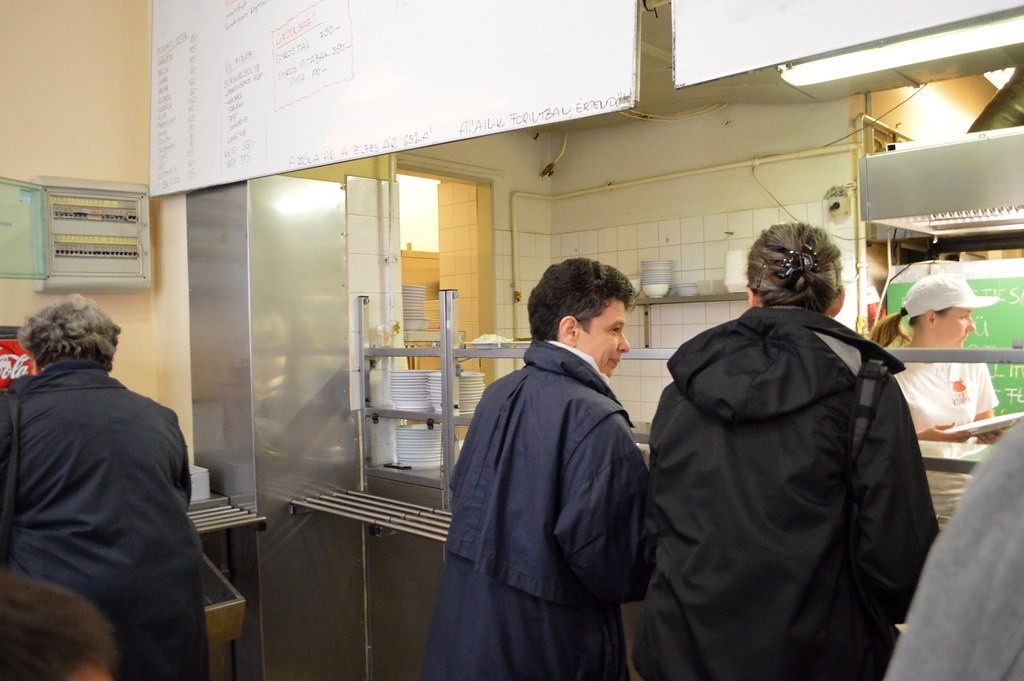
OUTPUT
[881,416,1024,681]
[631,222,940,681]
[0,572,117,681]
[419,257,653,681]
[0,291,210,681]
[870,273,1009,531]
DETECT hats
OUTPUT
[902,272,1001,318]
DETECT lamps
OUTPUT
[777,14,1024,86]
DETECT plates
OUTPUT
[500,341,531,348]
[395,424,442,469]
[640,260,673,297]
[402,285,431,318]
[944,412,1024,435]
[465,342,514,349]
[388,371,486,417]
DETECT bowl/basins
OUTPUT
[673,283,699,297]
[630,278,640,298]
[404,318,431,332]
[643,284,668,298]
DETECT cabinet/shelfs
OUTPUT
[357,288,1024,513]
